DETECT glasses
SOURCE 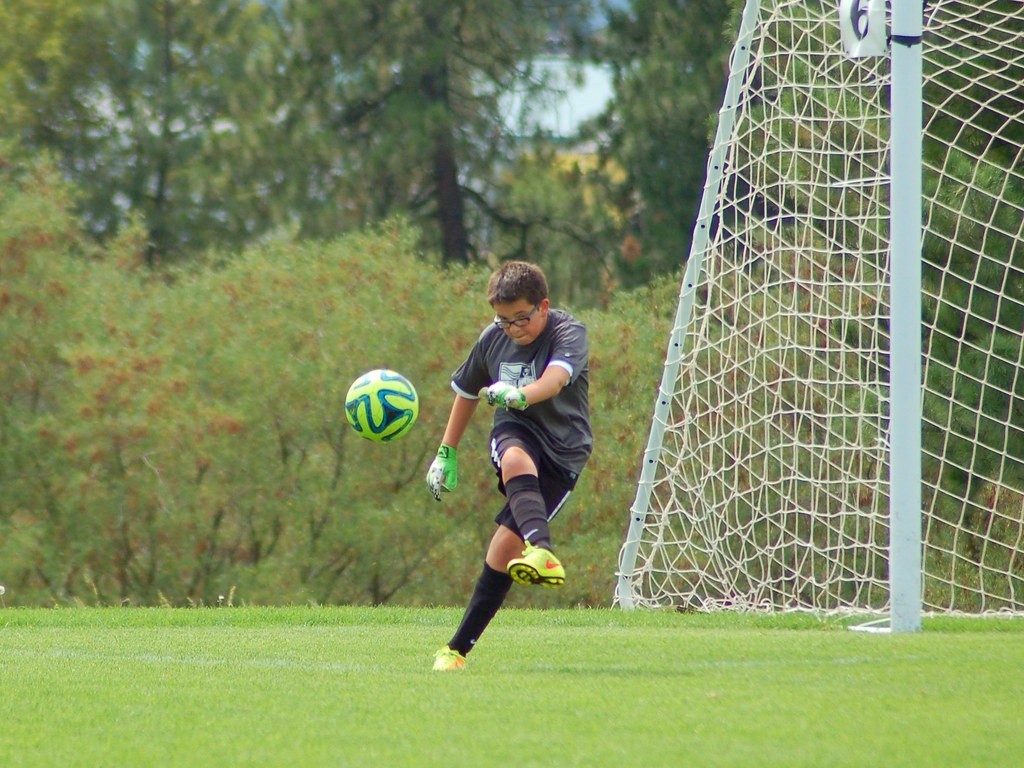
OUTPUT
[493,303,541,330]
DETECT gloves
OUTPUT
[478,381,530,412]
[425,442,458,501]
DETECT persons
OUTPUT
[424,261,595,670]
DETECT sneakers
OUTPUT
[506,540,566,588]
[432,645,467,671]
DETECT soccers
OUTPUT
[344,368,419,443]
